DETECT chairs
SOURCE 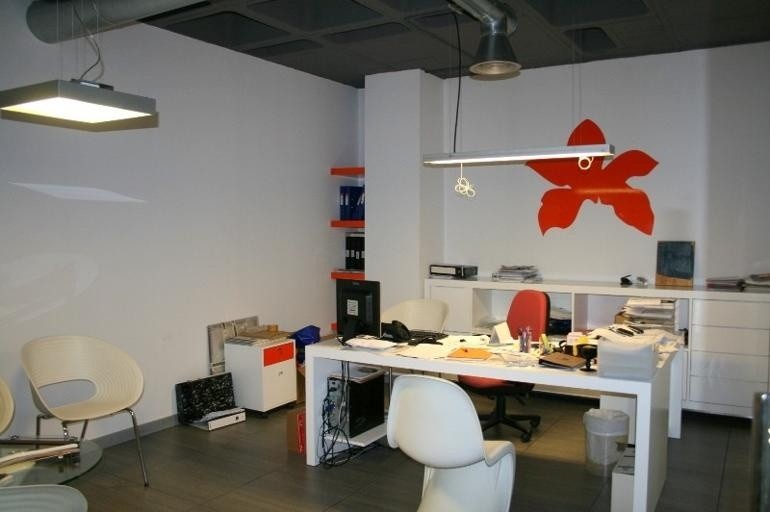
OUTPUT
[380,297,451,409]
[19,333,151,490]
[0,378,16,438]
[384,374,518,512]
[456,288,550,442]
[0,484,90,512]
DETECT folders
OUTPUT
[539,351,587,371]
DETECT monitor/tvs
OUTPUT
[335,279,382,345]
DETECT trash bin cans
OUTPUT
[583,409,629,479]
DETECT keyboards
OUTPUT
[408,329,449,341]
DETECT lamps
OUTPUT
[422,0,615,169]
[467,16,522,77]
[0,1,159,131]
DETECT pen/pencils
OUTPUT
[519,326,532,353]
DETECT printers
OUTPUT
[597,323,666,377]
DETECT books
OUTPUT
[656,241,696,286]
[624,297,674,332]
[705,275,770,295]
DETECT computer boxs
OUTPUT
[327,365,385,438]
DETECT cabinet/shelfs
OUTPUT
[223,338,298,420]
[423,277,770,419]
[326,166,365,332]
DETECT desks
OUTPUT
[302,334,686,512]
[0,434,103,487]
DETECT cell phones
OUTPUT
[629,325,644,334]
[617,328,634,337]
